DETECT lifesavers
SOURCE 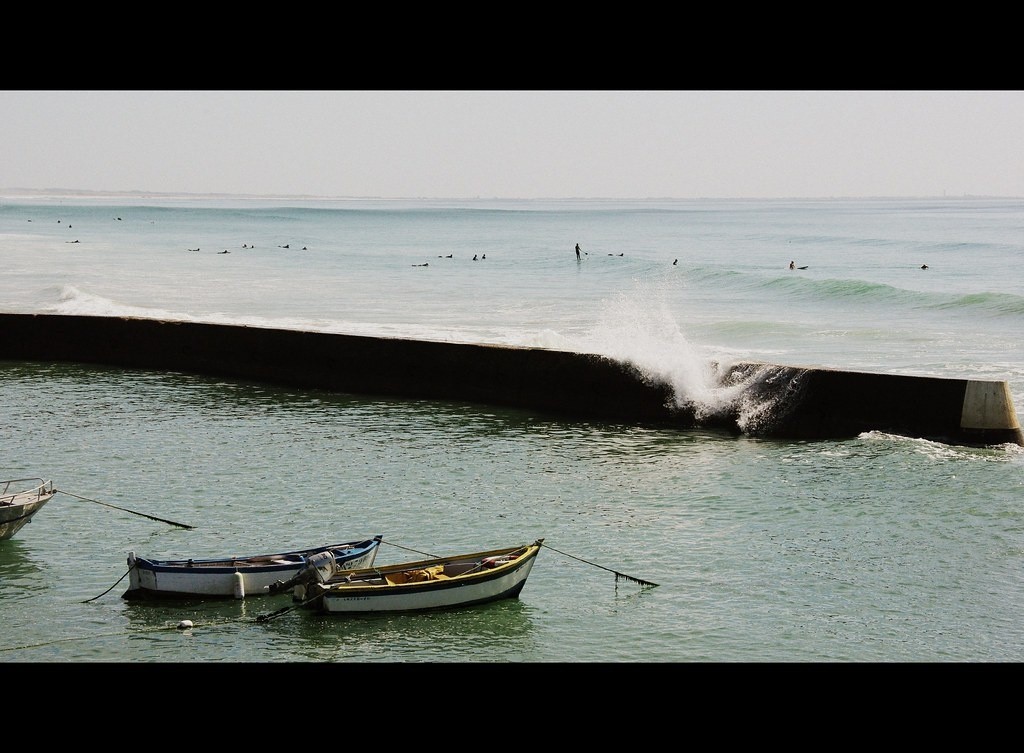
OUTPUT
[482,554,521,568]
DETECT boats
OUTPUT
[0,479,56,541]
[272,541,543,615]
[125,535,385,603]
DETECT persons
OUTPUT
[575,243,581,260]
